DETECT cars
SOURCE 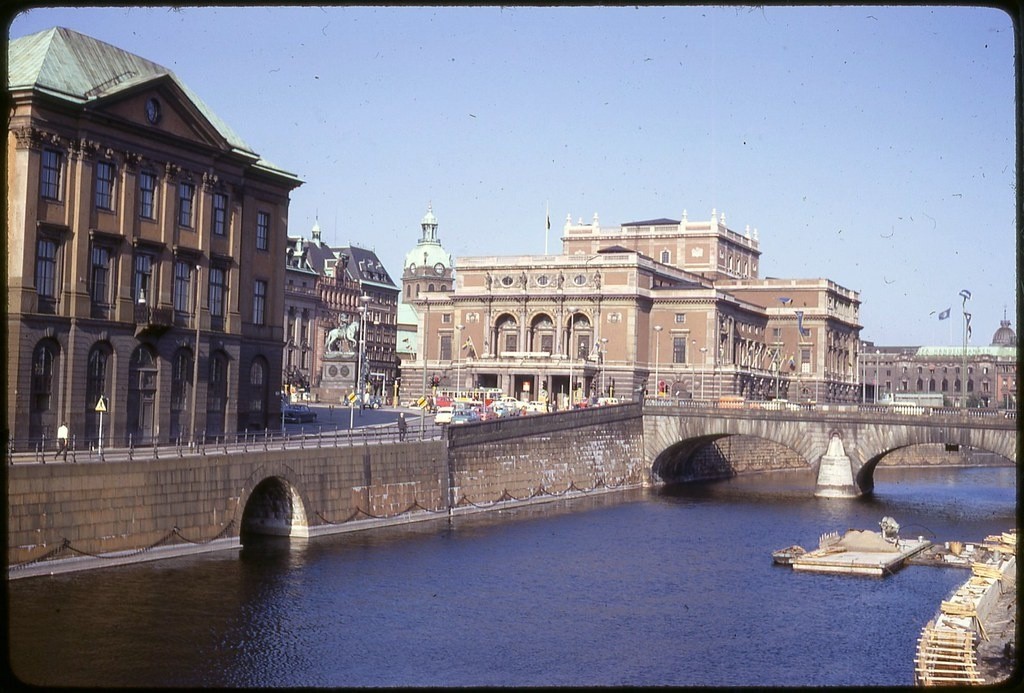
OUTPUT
[344,394,382,410]
[434,396,623,426]
[280,404,317,424]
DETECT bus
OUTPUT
[431,386,503,413]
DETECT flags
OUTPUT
[749,345,795,366]
[938,308,950,319]
[596,340,599,352]
[462,338,472,350]
[548,218,550,229]
[402,338,412,351]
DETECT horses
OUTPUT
[325,321,360,352]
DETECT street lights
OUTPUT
[567,306,579,410]
[863,342,867,403]
[356,306,366,395]
[652,325,663,401]
[359,295,373,416]
[699,347,708,399]
[455,324,465,398]
[601,338,608,397]
[875,349,880,403]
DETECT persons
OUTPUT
[398,412,407,442]
[427,396,432,414]
[55,422,68,461]
[385,388,392,405]
[553,401,557,411]
[364,392,372,410]
[522,406,526,415]
[510,409,519,416]
[340,313,348,328]
